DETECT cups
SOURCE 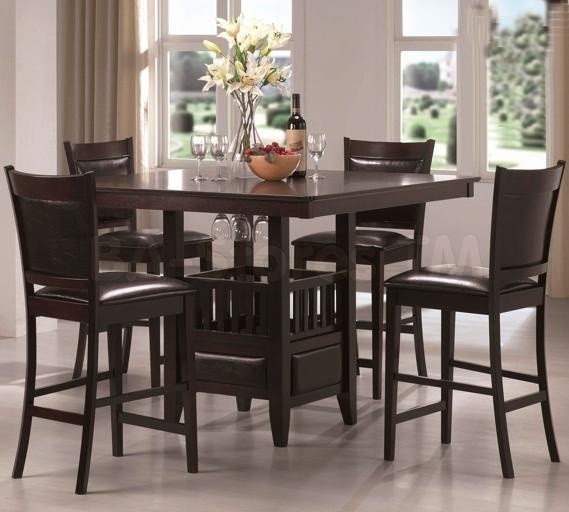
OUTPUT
[230,212,252,242]
[210,211,232,243]
[253,215,269,243]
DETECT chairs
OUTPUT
[382,158,565,478]
[63,135,217,387]
[2,165,201,494]
[291,136,436,398]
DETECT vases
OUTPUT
[225,93,268,178]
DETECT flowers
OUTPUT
[197,11,297,163]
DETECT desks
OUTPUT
[93,157,482,449]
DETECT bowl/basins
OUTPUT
[246,154,302,183]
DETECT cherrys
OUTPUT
[245,142,294,158]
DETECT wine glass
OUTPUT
[209,133,230,182]
[306,132,328,182]
[188,133,209,182]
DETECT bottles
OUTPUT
[285,93,307,179]
